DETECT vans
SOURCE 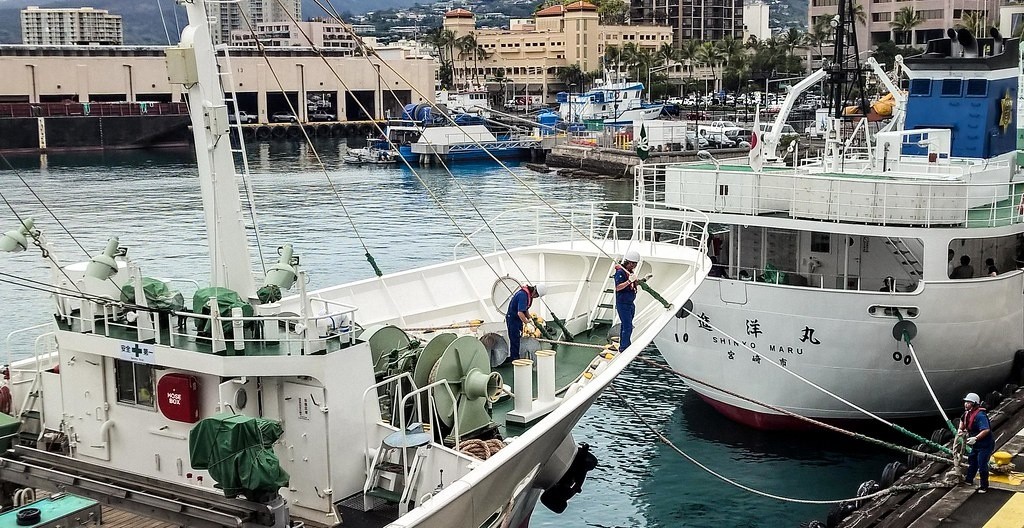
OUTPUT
[752,122,800,143]
[805,121,826,140]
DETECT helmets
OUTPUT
[535,284,547,298]
[962,393,980,404]
[624,251,640,262]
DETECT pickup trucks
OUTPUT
[307,110,336,122]
[698,120,743,137]
[726,109,756,122]
[228,110,258,124]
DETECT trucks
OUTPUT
[511,95,542,113]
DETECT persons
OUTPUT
[649,232,661,242]
[948,249,999,280]
[506,283,547,360]
[358,150,390,162]
[880,276,900,293]
[614,251,653,353]
[958,393,995,493]
[708,256,729,278]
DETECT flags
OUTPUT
[750,104,762,173]
[637,123,649,161]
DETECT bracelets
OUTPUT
[626,281,630,284]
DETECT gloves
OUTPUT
[628,273,637,283]
[966,436,977,445]
[644,272,653,281]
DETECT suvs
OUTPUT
[759,105,782,116]
[728,129,755,147]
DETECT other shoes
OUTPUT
[978,486,988,493]
[962,479,973,485]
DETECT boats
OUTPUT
[342,126,427,165]
[628,0,1023,426]
[1,1,713,528]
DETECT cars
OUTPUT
[503,100,513,111]
[654,92,883,106]
[686,130,709,150]
[793,105,812,113]
[271,110,298,123]
[704,133,736,150]
[686,110,711,120]
